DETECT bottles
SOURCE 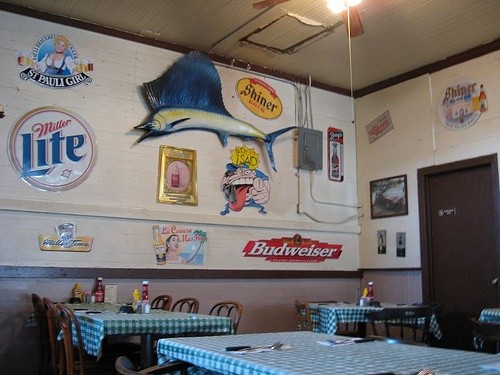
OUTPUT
[131,280,151,314]
[355,281,374,306]
[73,277,104,303]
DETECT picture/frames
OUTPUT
[370,174,409,219]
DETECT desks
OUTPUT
[22,301,234,370]
[473,308,500,349]
[299,302,444,340]
[155,331,500,375]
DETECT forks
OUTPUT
[225,340,281,350]
[416,368,431,375]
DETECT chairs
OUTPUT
[31,293,500,375]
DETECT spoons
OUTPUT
[239,343,284,351]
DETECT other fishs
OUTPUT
[134,50,300,171]
